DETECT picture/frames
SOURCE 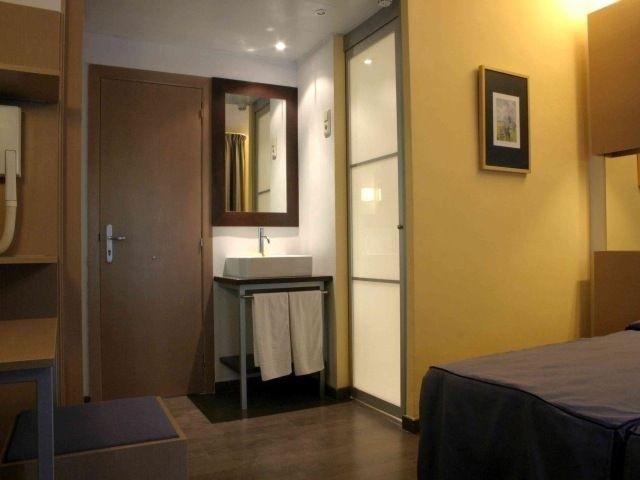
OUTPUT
[477,63,533,176]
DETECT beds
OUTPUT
[418,320,640,480]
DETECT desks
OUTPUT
[0,316,61,480]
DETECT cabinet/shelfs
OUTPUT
[212,275,333,411]
[583,0,640,338]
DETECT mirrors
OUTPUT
[210,76,300,229]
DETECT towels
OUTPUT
[251,289,326,382]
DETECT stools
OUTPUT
[0,395,191,480]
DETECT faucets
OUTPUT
[259,228,270,255]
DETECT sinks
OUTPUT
[226,255,312,279]
